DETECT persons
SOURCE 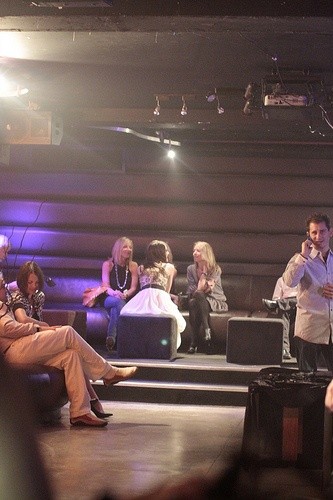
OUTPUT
[119,239,187,349]
[0,235,137,425]
[102,237,138,349]
[261,277,298,359]
[187,241,228,355]
[283,214,333,372]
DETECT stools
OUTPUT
[0,360,69,426]
[117,311,177,360]
[227,316,283,365]
[242,366,333,467]
[40,309,87,342]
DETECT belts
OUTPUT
[141,283,165,290]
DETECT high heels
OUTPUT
[90,397,113,418]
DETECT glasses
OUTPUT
[1,283,8,289]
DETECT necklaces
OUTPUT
[115,263,128,289]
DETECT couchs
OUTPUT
[5,267,283,355]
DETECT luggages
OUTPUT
[240,367,333,468]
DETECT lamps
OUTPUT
[243,82,261,116]
[154,96,161,115]
[214,89,224,114]
[180,95,188,116]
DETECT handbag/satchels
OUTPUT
[82,286,108,308]
[178,292,189,311]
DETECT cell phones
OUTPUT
[307,237,311,241]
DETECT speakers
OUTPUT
[42,310,86,337]
[226,317,290,365]
[117,314,178,360]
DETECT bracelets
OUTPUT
[201,271,207,275]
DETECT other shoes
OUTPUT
[187,340,195,354]
[204,327,211,341]
[69,409,109,427]
[261,298,279,312]
[282,349,293,359]
[106,335,115,352]
[102,366,137,387]
